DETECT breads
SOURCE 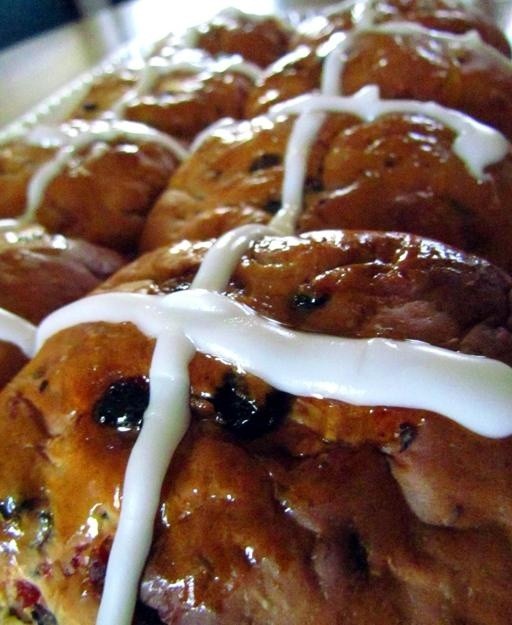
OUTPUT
[0,0,512,625]
